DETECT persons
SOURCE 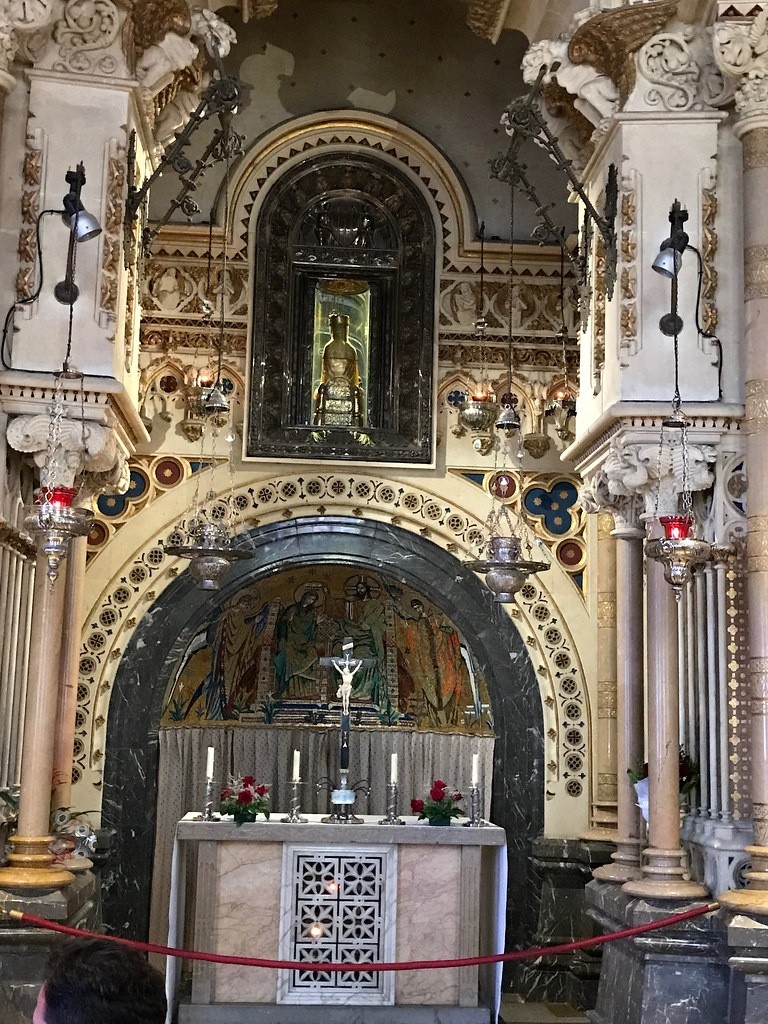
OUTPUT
[330,660,363,716]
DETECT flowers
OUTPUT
[218,772,273,828]
[410,780,466,823]
[627,743,699,827]
[0,768,101,832]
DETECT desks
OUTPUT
[165,812,508,1024]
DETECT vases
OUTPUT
[234,812,258,822]
[429,818,450,826]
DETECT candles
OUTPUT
[206,747,214,777]
[293,749,300,778]
[472,754,479,781]
[391,752,397,782]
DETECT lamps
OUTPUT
[21,159,93,595]
[651,197,689,337]
[544,226,577,438]
[462,177,550,603]
[646,201,712,605]
[460,220,497,453]
[54,170,102,304]
[163,112,255,591]
